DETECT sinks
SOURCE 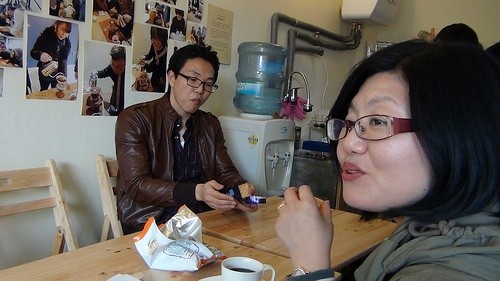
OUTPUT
[302,141,331,153]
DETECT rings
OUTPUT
[276,203,285,213]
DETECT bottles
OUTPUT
[233,42,287,115]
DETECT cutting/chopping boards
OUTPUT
[99,18,124,43]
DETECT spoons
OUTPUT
[104,20,115,32]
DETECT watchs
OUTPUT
[291,266,309,277]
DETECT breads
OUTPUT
[238,182,255,198]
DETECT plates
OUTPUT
[199,274,265,281]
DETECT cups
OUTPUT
[221,257,276,281]
[57,75,67,87]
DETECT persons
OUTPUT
[0,6,24,67]
[30,20,73,91]
[433,23,500,68]
[273,38,500,281]
[115,42,258,235]
[89,45,126,116]
[94,0,207,42]
[138,26,168,93]
[50,0,81,21]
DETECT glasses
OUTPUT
[109,60,124,70]
[324,114,415,141]
[172,67,219,93]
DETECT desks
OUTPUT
[0,197,398,281]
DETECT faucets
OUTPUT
[282,71,313,112]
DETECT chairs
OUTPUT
[0,155,124,269]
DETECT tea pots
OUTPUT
[40,60,63,78]
